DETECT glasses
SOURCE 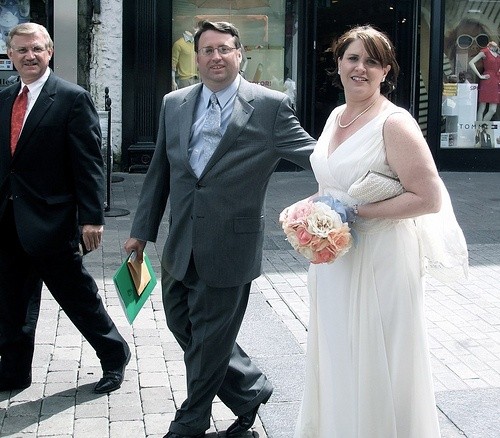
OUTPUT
[196,45,238,55]
[455,34,489,50]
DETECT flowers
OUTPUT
[280,200,354,265]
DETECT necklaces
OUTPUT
[338,100,375,128]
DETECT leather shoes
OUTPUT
[226,389,273,438]
[163,431,205,438]
[93,351,131,394]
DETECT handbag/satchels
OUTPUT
[347,170,406,203]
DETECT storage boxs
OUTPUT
[440,132,454,146]
[475,121,500,147]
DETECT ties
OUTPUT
[195,94,221,179]
[10,85,29,157]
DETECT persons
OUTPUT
[294,25,443,438]
[469,41,500,121]
[171,31,202,91]
[0,22,131,394]
[124,21,317,438]
[443,11,500,84]
[475,123,491,147]
